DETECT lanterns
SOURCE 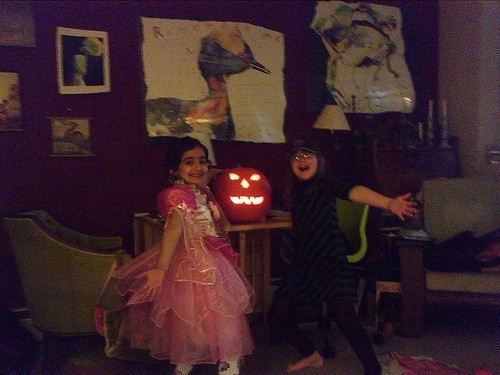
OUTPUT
[211,164,272,224]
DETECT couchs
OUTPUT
[2,207,153,375]
[395,178,499,340]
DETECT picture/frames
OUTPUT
[55,26,112,95]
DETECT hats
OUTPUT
[289,140,321,156]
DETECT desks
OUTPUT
[133,208,293,330]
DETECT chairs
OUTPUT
[270,197,384,359]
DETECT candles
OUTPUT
[418,94,453,148]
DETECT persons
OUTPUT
[110,136,255,374]
[268,141,417,375]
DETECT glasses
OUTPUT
[290,150,314,162]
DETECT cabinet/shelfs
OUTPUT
[370,137,460,280]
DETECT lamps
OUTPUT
[214,162,273,225]
[312,104,351,137]
